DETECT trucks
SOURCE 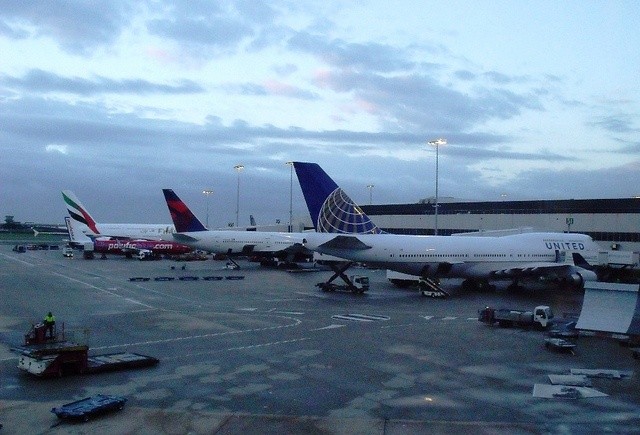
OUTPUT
[543,329,576,354]
[63,247,74,257]
[494,305,554,330]
[315,275,370,293]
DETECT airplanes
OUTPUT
[294,161,601,294]
[61,189,195,259]
[155,162,312,272]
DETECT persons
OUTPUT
[44,312,56,337]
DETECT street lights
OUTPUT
[366,185,374,205]
[233,165,244,226]
[285,162,294,226]
[428,139,447,235]
[203,190,214,227]
